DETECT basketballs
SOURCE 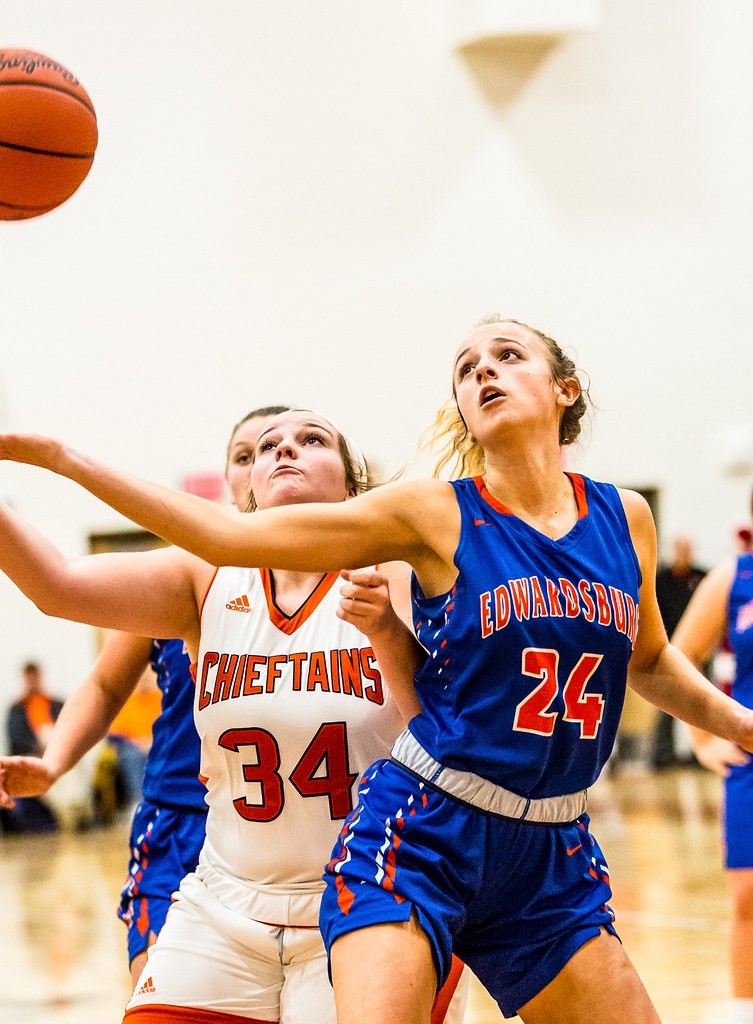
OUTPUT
[1,45,98,220]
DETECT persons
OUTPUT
[647,536,716,774]
[106,663,162,803]
[0,407,472,1024]
[670,482,753,1002]
[5,660,65,758]
[0,406,291,998]
[0,310,753,1024]
